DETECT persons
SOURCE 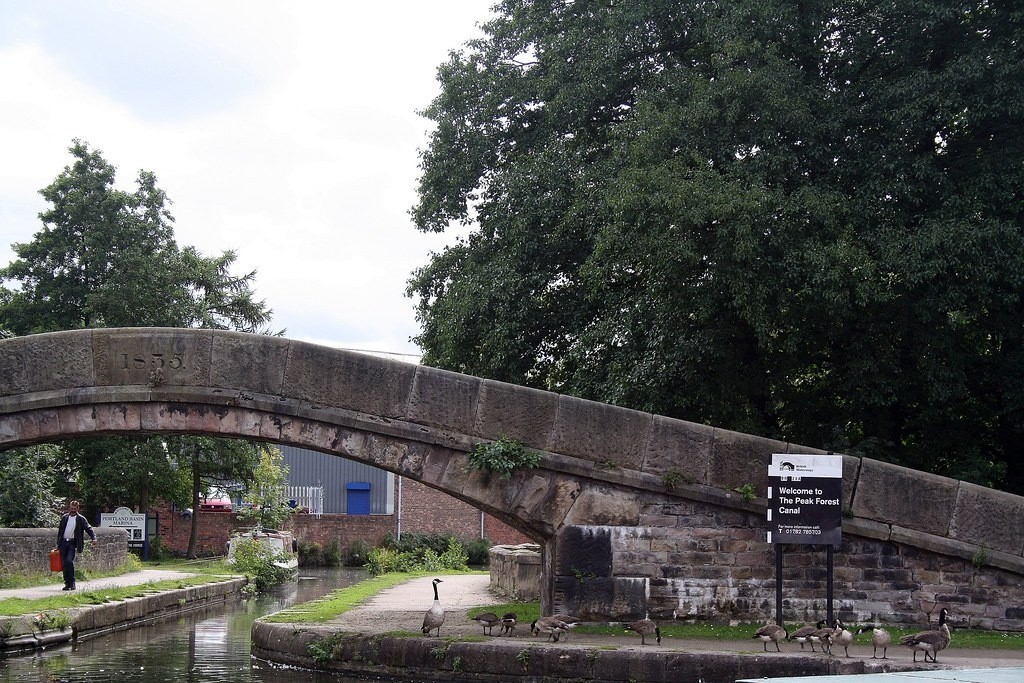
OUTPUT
[58,501,97,593]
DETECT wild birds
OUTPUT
[898,608,952,664]
[498,612,517,637]
[853,625,892,659]
[530,612,584,643]
[621,619,661,647]
[751,625,797,652]
[787,619,854,659]
[421,579,446,638]
[470,612,501,638]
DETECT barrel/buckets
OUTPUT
[50,548,63,572]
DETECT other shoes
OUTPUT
[63,585,69,590]
[71,586,76,589]
